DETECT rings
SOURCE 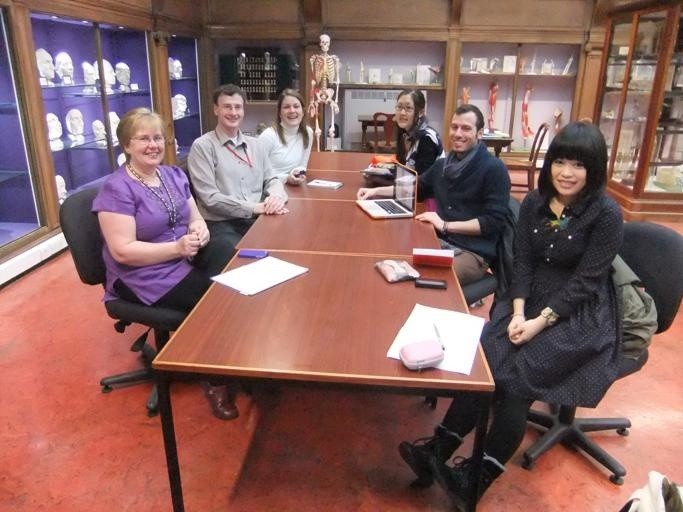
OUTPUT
[205,237,210,242]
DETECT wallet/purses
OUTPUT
[398,337,448,372]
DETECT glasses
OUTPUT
[393,103,416,113]
[128,134,167,144]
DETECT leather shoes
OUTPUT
[205,384,240,421]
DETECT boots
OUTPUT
[428,451,507,512]
[398,423,465,489]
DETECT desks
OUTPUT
[150,150,496,511]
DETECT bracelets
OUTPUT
[439,220,448,235]
[510,313,525,319]
[539,306,559,327]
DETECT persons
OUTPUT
[90,107,240,421]
[355,103,512,288]
[184,82,289,249]
[397,119,625,512]
[257,86,314,188]
[392,86,444,178]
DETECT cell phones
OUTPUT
[238,248,268,259]
[415,278,449,290]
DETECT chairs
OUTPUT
[58,173,203,416]
[522,220,682,485]
[461,195,521,304]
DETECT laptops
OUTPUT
[355,160,418,220]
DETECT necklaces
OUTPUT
[126,164,178,242]
